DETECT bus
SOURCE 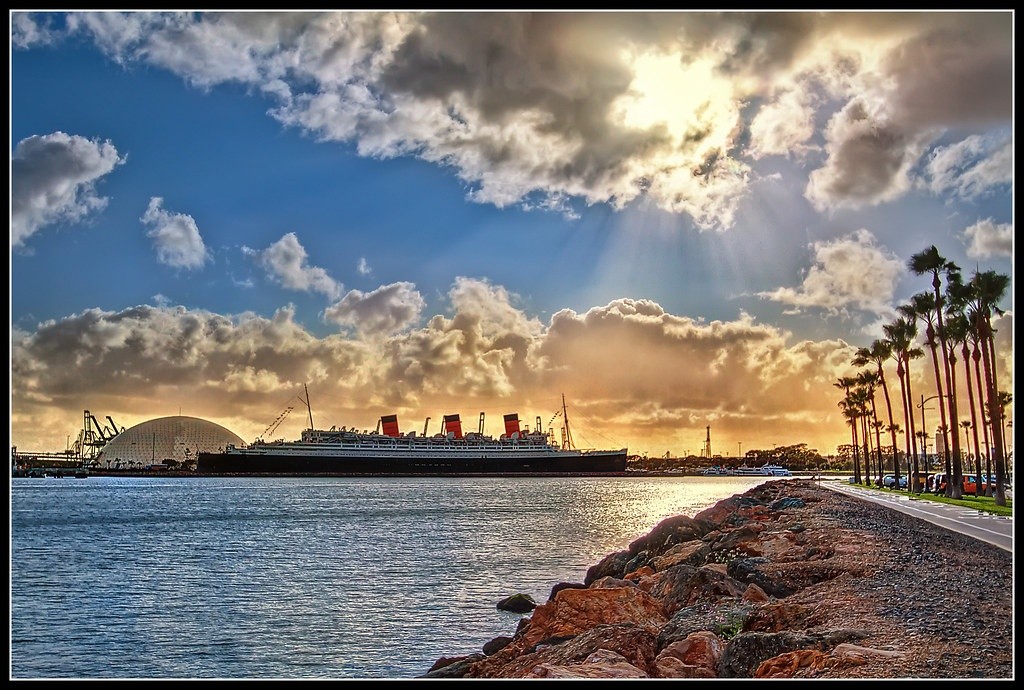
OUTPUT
[145,464,168,470]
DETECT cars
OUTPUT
[873,471,1011,496]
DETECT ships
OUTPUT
[196,382,628,474]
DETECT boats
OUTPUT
[708,460,788,476]
[12,465,88,479]
